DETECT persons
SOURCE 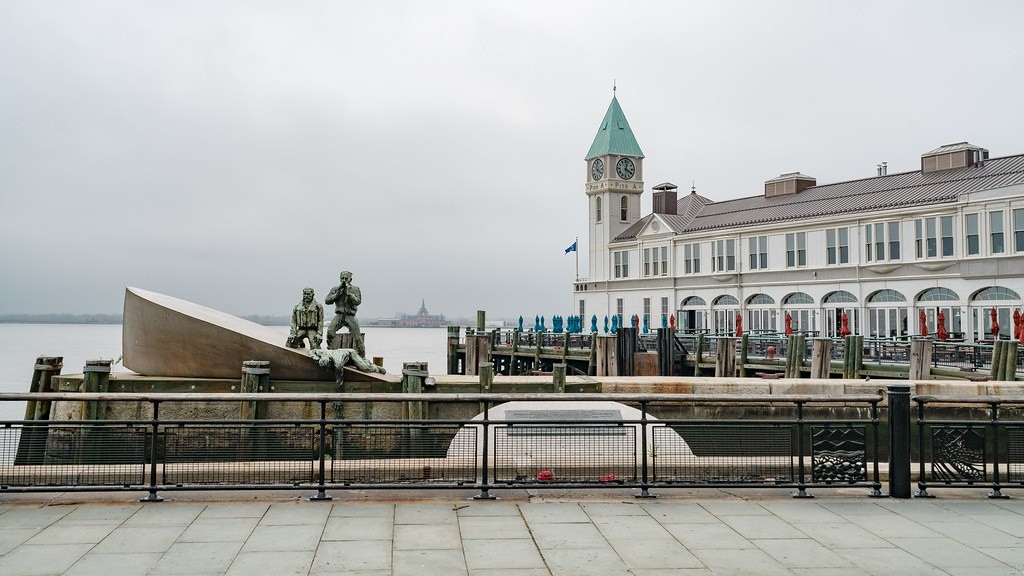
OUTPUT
[287,288,324,350]
[325,271,367,360]
[308,349,386,410]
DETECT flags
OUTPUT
[565,241,577,255]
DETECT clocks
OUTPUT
[616,158,635,180]
[592,159,604,181]
